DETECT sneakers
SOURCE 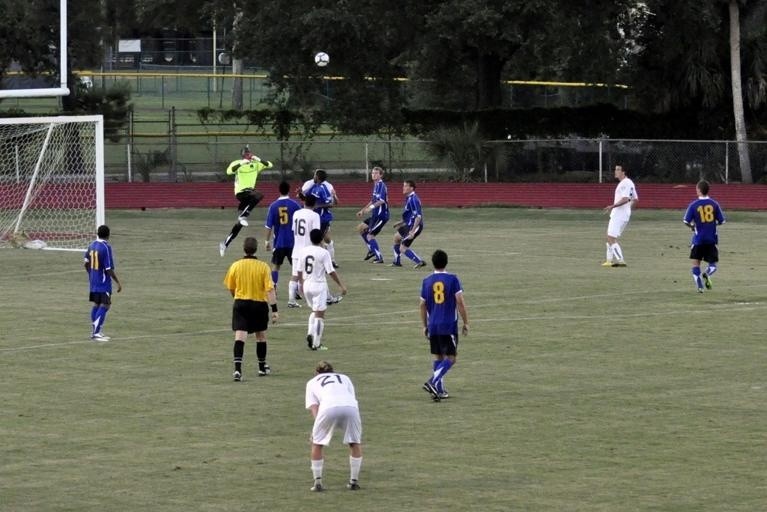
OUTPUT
[238,215,248,227]
[364,251,375,260]
[702,273,713,289]
[612,262,627,267]
[346,478,359,489]
[296,293,302,299]
[233,371,242,381]
[312,344,328,351]
[100,333,110,340]
[288,302,302,308]
[385,262,402,267]
[89,335,108,341]
[332,260,338,268]
[311,479,323,491]
[220,242,226,257]
[307,334,313,348]
[259,366,270,375]
[326,296,343,305]
[602,262,613,266]
[422,382,440,402]
[412,261,426,269]
[697,288,704,293]
[368,259,384,264]
[439,391,448,398]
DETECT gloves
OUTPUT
[240,159,249,165]
[251,156,261,162]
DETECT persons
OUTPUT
[219,146,274,258]
[356,167,390,264]
[601,163,638,267]
[298,229,346,350]
[302,177,338,268]
[222,237,279,382]
[265,181,302,300]
[305,362,363,491]
[386,180,427,269]
[682,180,726,293]
[84,225,122,343]
[420,249,469,403]
[300,169,330,257]
[286,195,321,307]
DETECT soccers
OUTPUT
[315,52,329,67]
